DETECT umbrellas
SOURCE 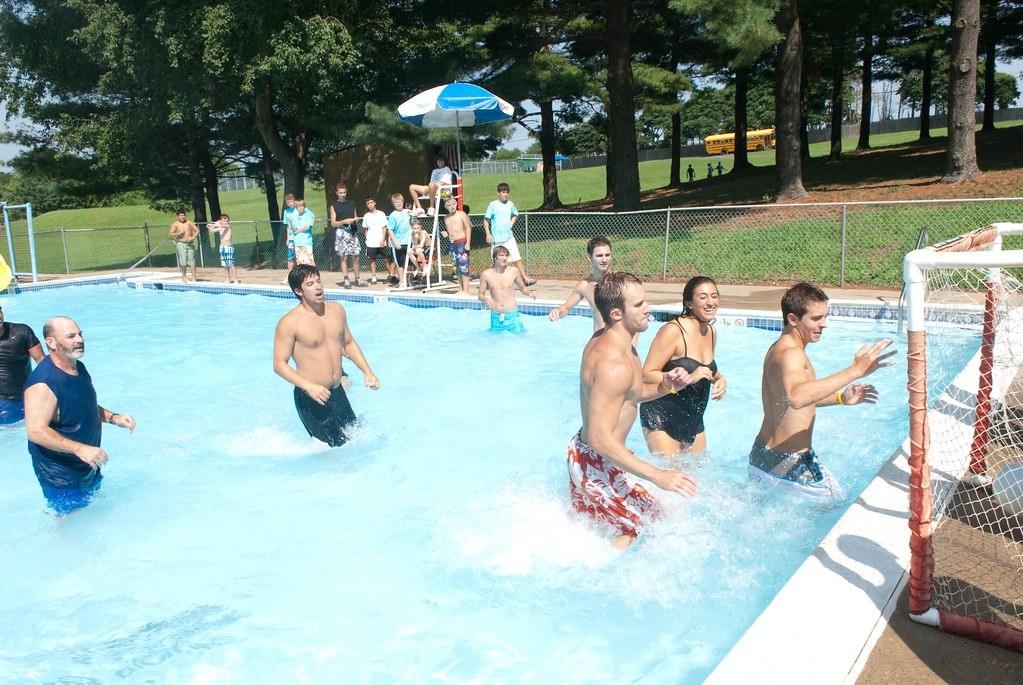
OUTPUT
[397,80,514,177]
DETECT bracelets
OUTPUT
[660,383,677,395]
[837,392,844,406]
[109,414,119,425]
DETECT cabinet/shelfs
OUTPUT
[390,172,462,292]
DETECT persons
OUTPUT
[273,264,381,447]
[168,209,201,283]
[24,317,136,514]
[0,306,45,426]
[547,236,640,349]
[483,183,537,287]
[640,276,727,457]
[280,194,315,285]
[387,193,473,297]
[748,282,898,500]
[686,161,727,181]
[206,214,240,285]
[477,246,537,335]
[362,196,390,285]
[330,183,369,289]
[565,271,698,547]
[409,153,453,216]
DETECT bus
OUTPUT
[704,127,776,155]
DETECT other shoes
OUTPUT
[344,280,352,289]
[410,208,425,216]
[523,280,536,287]
[388,276,395,285]
[370,277,377,285]
[354,279,369,287]
[427,208,435,216]
[281,280,291,286]
